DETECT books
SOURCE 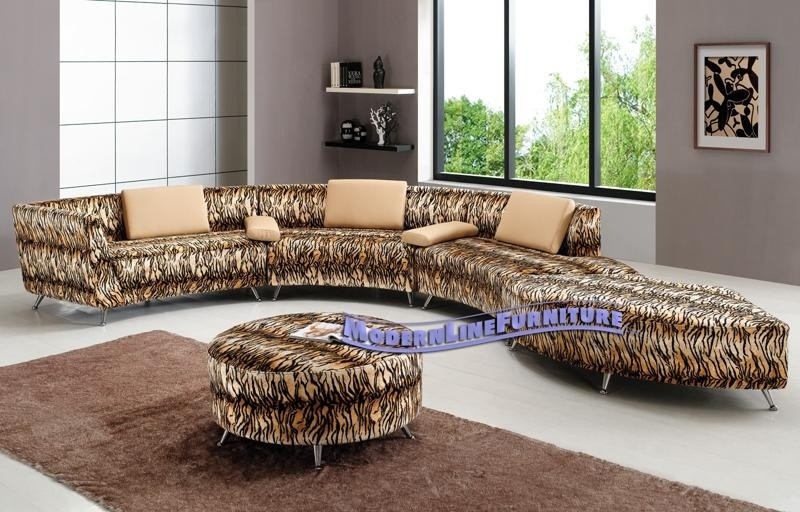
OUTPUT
[329,61,364,89]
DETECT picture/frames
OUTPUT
[693,40,771,153]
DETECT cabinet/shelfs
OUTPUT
[325,85,415,153]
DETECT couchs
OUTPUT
[14,178,789,412]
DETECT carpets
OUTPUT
[1,330,779,512]
